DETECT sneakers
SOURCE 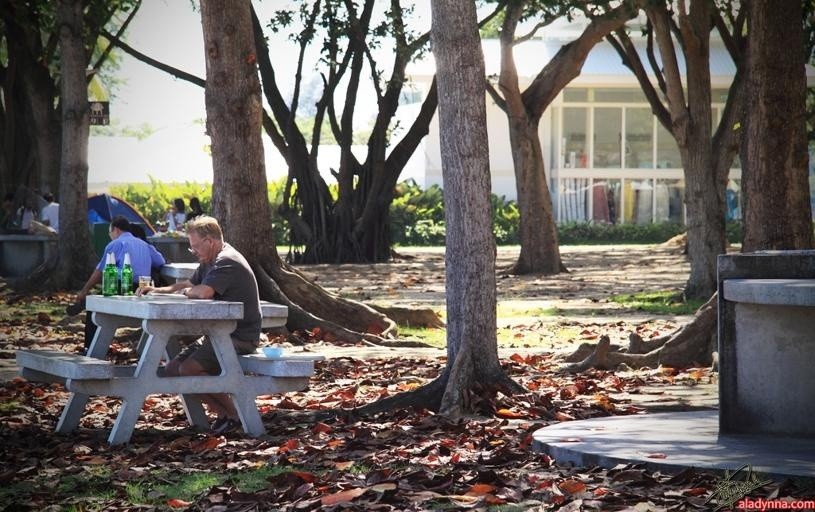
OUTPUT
[211,414,228,433]
[221,419,242,435]
[65,303,84,316]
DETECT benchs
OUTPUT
[8,349,115,399]
[231,352,317,392]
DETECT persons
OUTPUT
[66,214,167,359]
[0,191,206,295]
[134,212,264,433]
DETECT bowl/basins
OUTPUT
[261,347,285,359]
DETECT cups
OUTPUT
[154,220,188,237]
[138,275,152,288]
[176,278,187,294]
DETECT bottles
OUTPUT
[102,251,118,296]
[121,252,133,296]
